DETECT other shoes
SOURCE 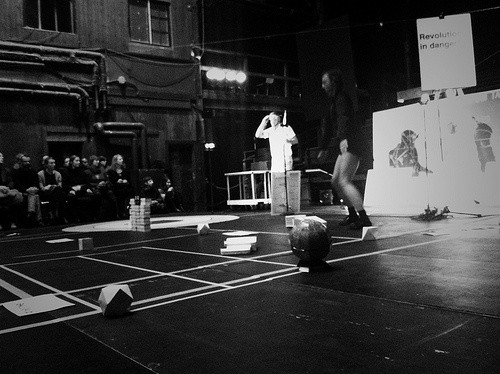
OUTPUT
[350,215,372,228]
[338,215,359,225]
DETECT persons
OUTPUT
[317,70,373,231]
[0,151,186,237]
[255,110,298,215]
